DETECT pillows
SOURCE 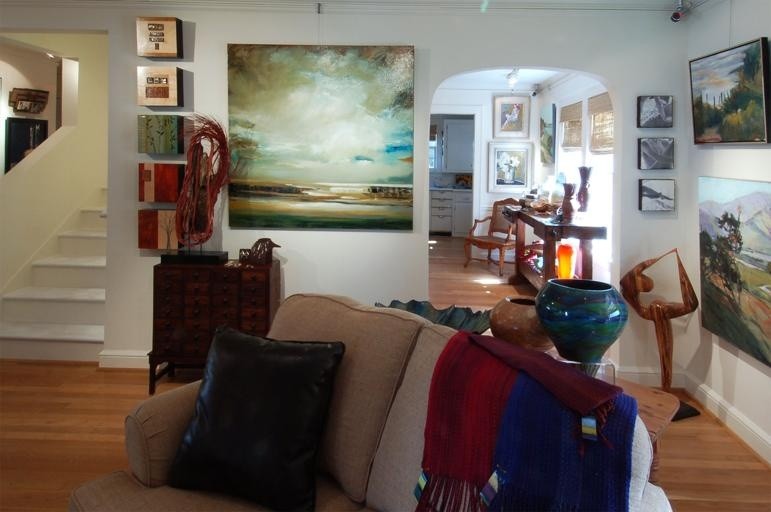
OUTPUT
[167,328,346,511]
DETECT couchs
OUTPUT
[66,292,674,512]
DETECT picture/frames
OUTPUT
[689,36,771,145]
[493,96,529,139]
[5,117,48,173]
[488,141,532,194]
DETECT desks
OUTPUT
[501,202,607,292]
[594,371,681,485]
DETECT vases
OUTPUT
[534,278,629,377]
[489,294,556,352]
[561,183,576,219]
[576,166,592,212]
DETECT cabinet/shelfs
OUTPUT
[431,190,453,233]
[452,191,473,237]
[146,255,281,395]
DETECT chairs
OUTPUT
[463,197,520,276]
